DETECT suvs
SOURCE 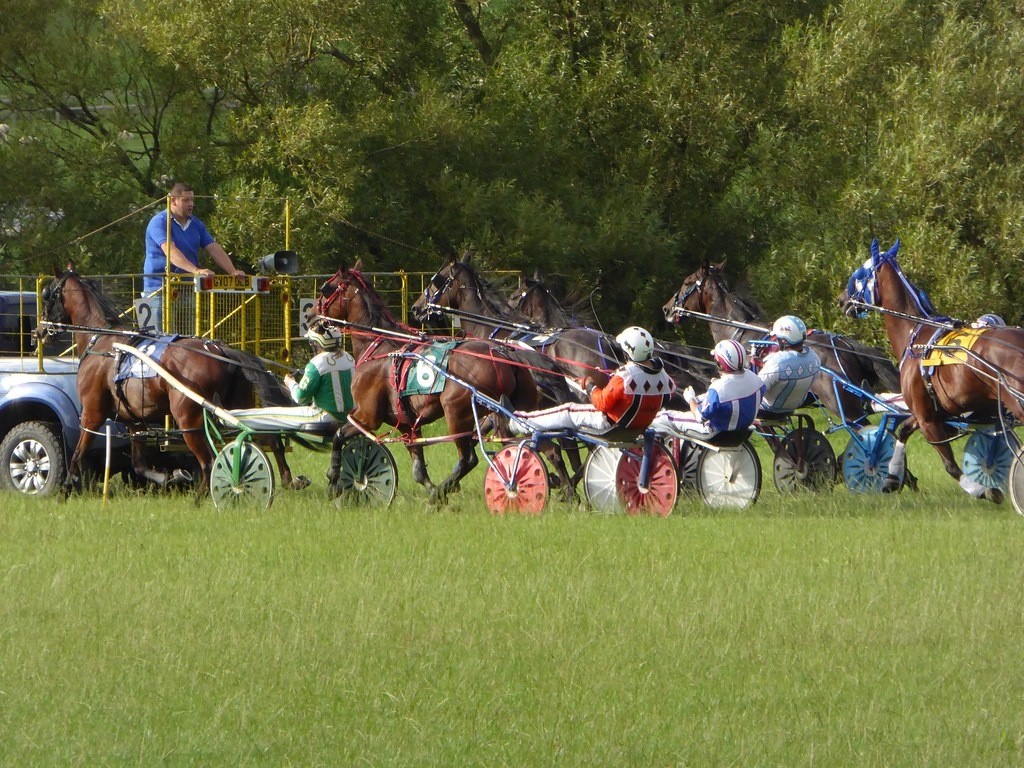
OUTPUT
[0,290,211,502]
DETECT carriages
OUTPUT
[408,251,839,516]
[662,260,1018,497]
[31,261,399,519]
[300,257,682,523]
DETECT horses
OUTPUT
[837,237,1024,505]
[662,259,921,496]
[303,252,637,516]
[31,258,311,507]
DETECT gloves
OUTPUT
[711,377,716,384]
[683,385,695,402]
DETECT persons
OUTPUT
[696,316,822,416]
[860,315,1003,415]
[212,318,359,429]
[145,183,247,336]
[498,327,678,436]
[645,340,767,439]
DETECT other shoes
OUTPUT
[213,392,224,428]
[501,394,517,437]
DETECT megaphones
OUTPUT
[260,251,298,274]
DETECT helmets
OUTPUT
[770,314,807,344]
[971,313,1006,329]
[616,327,654,360]
[709,340,744,371]
[303,318,342,350]
